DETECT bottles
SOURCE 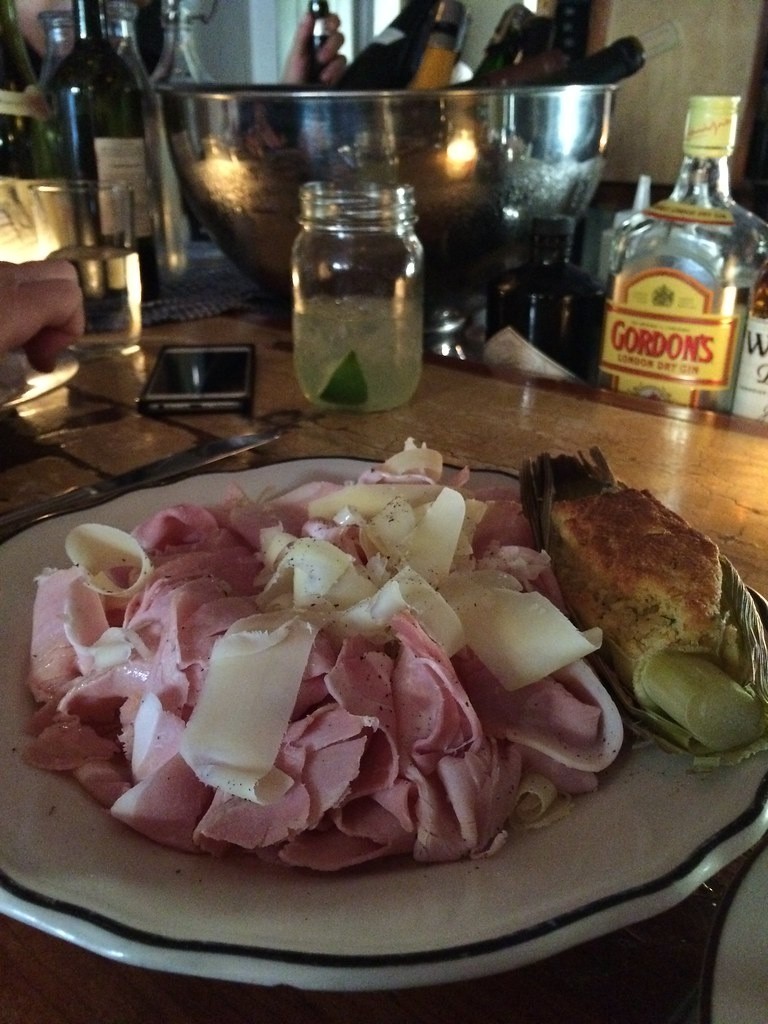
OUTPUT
[309,0,685,89]
[486,214,603,371]
[594,97,767,413]
[0,0,225,304]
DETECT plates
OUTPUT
[0,455,766,991]
[699,840,768,1023]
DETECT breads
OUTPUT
[547,483,723,656]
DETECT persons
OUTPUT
[0,260,87,375]
[0,0,346,89]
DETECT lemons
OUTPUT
[316,350,367,405]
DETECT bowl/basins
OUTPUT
[159,84,620,315]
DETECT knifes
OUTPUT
[1,423,299,525]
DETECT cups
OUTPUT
[292,179,424,412]
[27,180,142,347]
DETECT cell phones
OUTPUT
[137,342,257,417]
